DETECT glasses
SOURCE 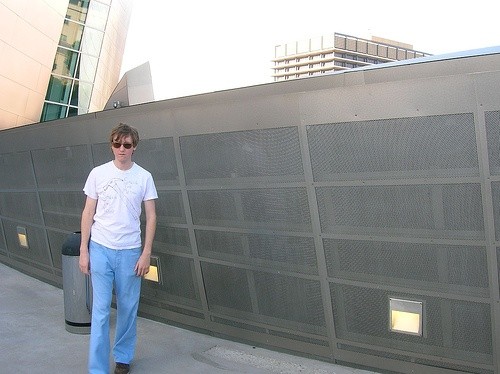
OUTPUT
[112,141,133,149]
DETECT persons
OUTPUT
[79,122,159,374]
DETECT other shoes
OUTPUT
[115,362,130,374]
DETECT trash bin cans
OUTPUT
[62,232,93,335]
